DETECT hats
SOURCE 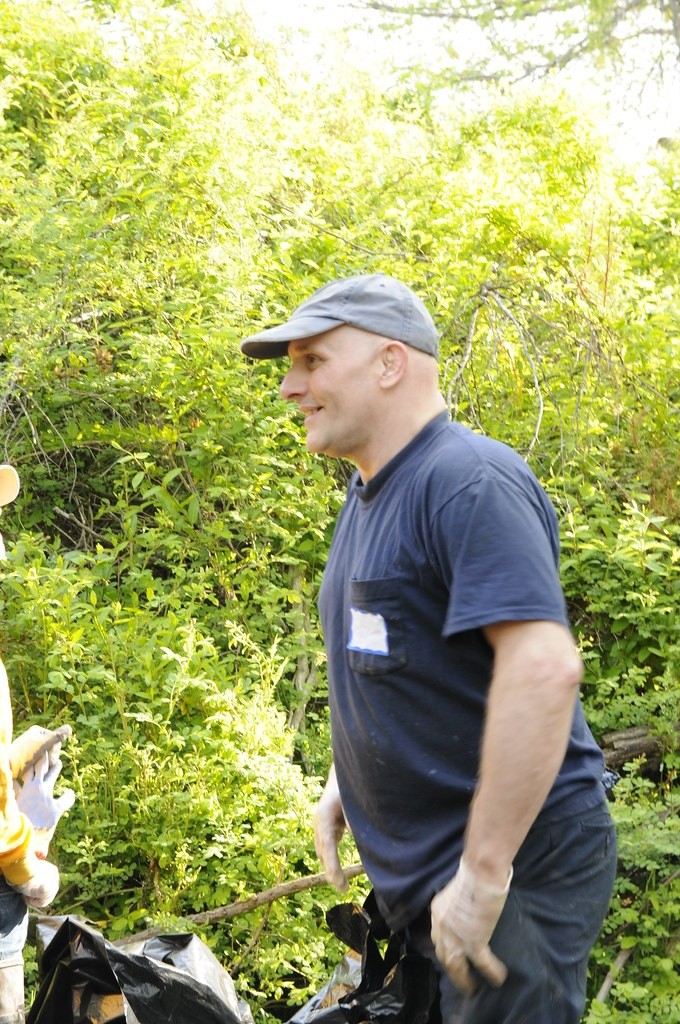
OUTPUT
[0,464,21,506]
[240,273,439,362]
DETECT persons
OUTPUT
[0,462,77,1022]
[242,273,618,1024]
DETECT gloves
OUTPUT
[314,760,353,894]
[12,740,77,860]
[9,724,73,782]
[430,854,513,995]
[5,856,60,908]
